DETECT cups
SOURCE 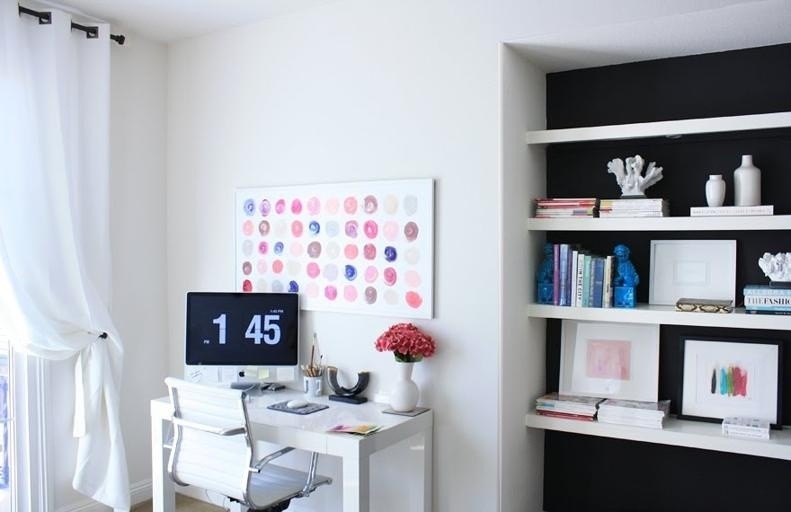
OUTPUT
[303,374,323,397]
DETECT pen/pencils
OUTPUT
[300,355,326,376]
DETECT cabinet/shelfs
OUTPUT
[523,111,791,462]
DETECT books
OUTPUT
[528,192,672,220]
[535,391,675,432]
[688,204,776,218]
[741,281,791,316]
[722,416,772,441]
[551,242,617,308]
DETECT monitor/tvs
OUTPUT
[187,292,299,383]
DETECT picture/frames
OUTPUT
[649,239,738,306]
[558,319,660,403]
[677,335,784,431]
[234,177,435,321]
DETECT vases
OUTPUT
[390,362,419,412]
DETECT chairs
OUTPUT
[164,377,332,512]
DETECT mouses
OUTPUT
[287,399,308,408]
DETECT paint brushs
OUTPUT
[310,334,316,368]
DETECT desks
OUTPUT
[150,383,434,512]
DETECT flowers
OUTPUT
[374,322,437,363]
